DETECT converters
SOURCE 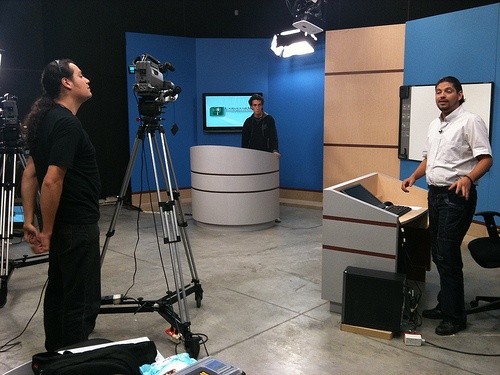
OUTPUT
[101,294,123,304]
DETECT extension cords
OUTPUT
[403,333,422,346]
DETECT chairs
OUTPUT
[464,211,500,323]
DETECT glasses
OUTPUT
[54,59,67,78]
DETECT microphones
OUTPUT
[166,63,175,72]
[439,130,442,132]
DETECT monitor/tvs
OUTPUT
[10,203,25,226]
[340,184,386,209]
[203,92,263,133]
[398,82,494,162]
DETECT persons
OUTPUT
[241,94,280,159]
[20,58,99,351]
[401,74,493,335]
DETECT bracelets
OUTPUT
[463,175,474,184]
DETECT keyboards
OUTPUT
[385,206,411,216]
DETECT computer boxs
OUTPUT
[341,266,406,336]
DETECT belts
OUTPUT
[428,185,477,192]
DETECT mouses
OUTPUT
[383,201,393,206]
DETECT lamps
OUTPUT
[271,0,327,57]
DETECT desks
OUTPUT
[323,171,431,318]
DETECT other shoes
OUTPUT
[422,302,447,320]
[435,315,468,336]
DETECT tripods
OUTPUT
[99,116,203,359]
[0,145,41,280]
